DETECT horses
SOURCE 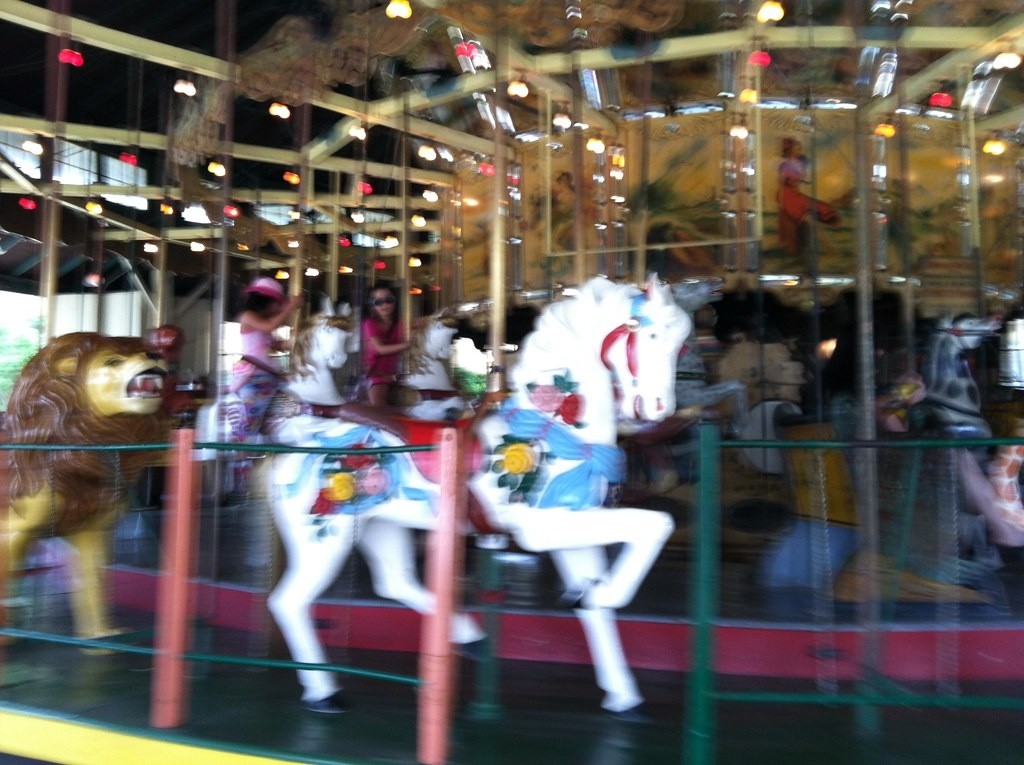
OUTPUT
[187,268,1024,738]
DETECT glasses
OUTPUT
[371,295,396,306]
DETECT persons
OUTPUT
[225,276,306,481]
[361,280,417,409]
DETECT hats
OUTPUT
[243,277,285,303]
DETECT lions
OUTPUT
[1,332,177,661]
